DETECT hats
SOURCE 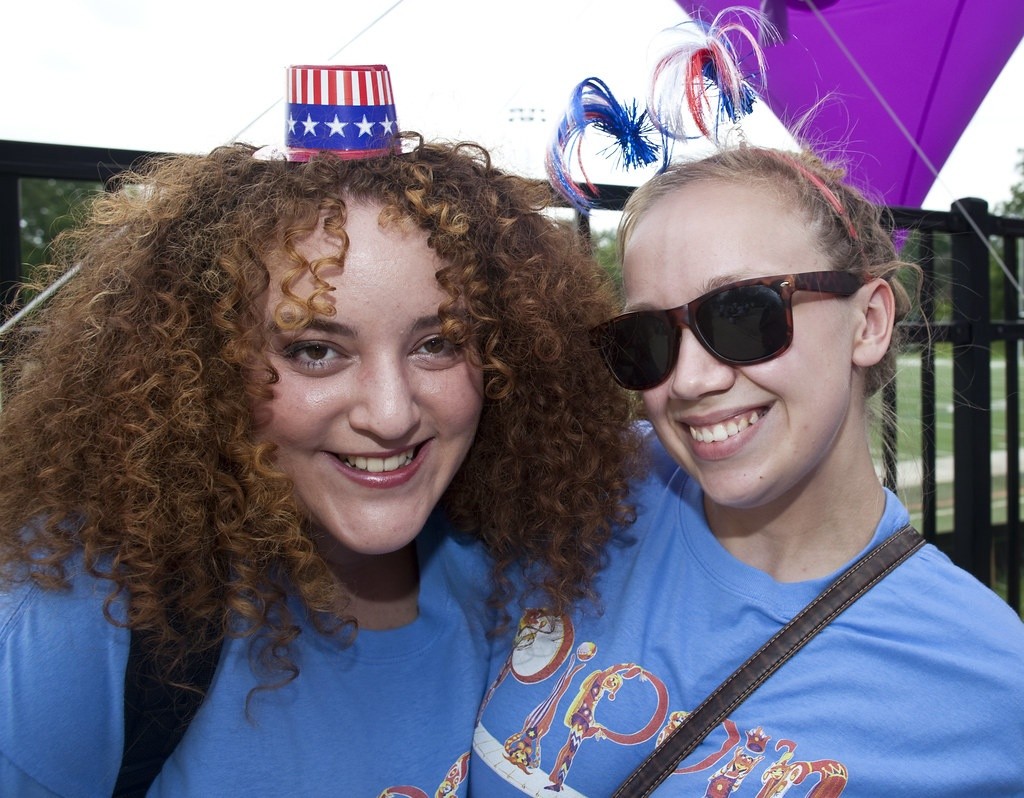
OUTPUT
[251,65,414,163]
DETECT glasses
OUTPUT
[586,269,863,391]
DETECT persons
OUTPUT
[466,146,1024,798]
[0,131,647,798]
